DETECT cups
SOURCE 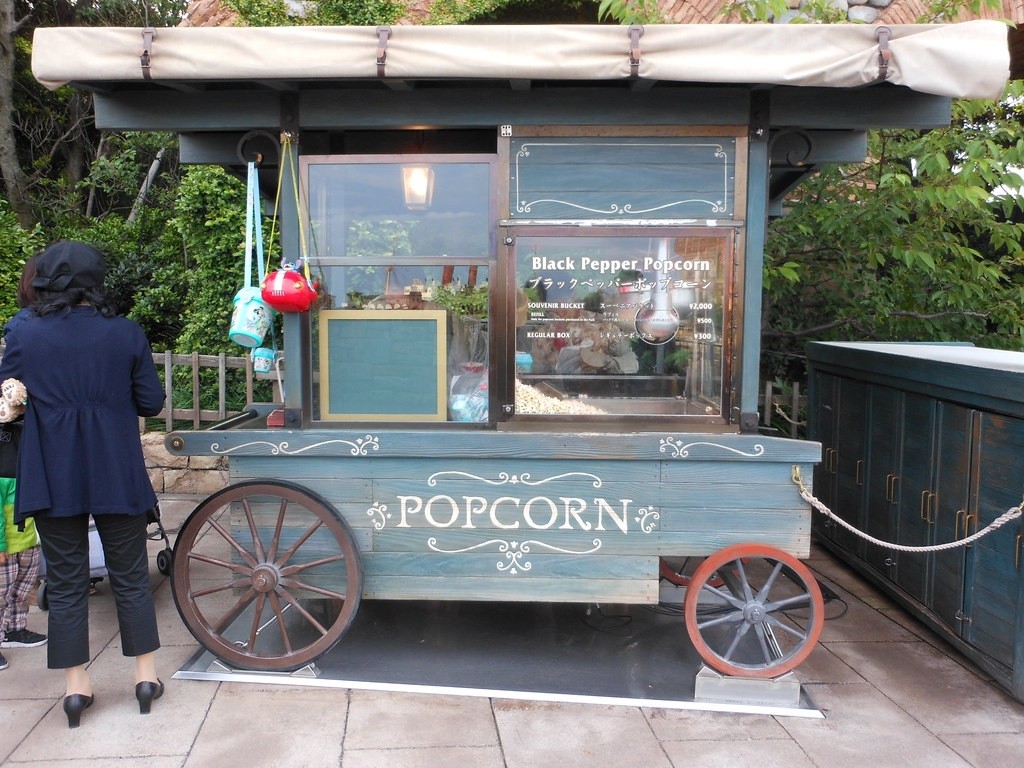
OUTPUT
[516,353,533,374]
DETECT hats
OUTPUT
[30,241,106,292]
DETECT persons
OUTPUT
[516,270,640,397]
[0,239,166,729]
[0,478,47,670]
[364,265,469,392]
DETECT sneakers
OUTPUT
[0,652,8,670]
[1,629,49,648]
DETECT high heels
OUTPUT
[136,678,164,714]
[64,694,94,729]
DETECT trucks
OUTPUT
[31,20,1011,679]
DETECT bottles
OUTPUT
[227,286,277,348]
[252,346,274,373]
